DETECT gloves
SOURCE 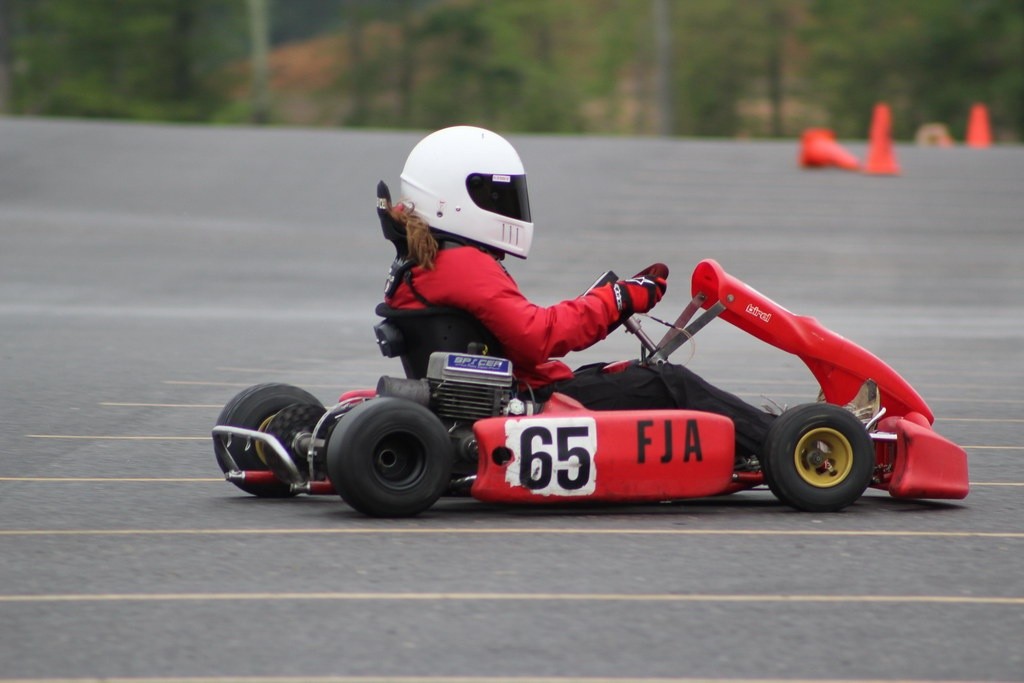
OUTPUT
[614,261,670,315]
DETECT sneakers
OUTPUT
[840,376,881,431]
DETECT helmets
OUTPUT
[398,124,535,261]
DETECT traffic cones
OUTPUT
[864,102,901,177]
[966,104,993,148]
[799,127,861,169]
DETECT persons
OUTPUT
[385,126,882,469]
